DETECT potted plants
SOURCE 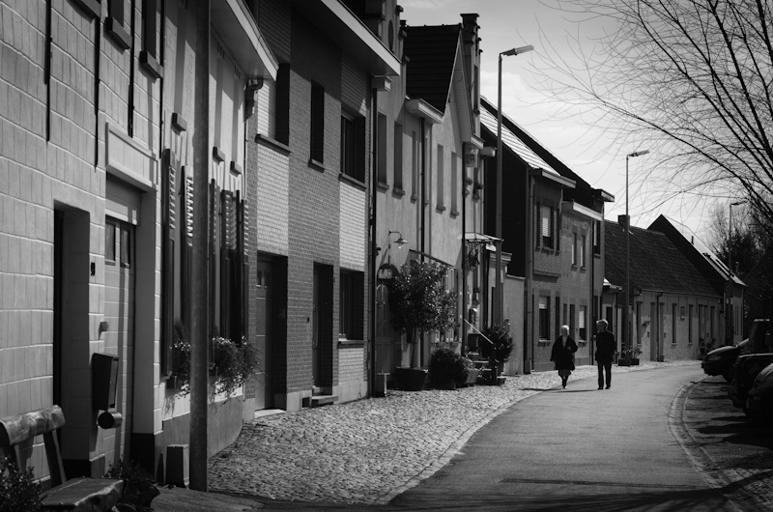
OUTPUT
[387,258,461,389]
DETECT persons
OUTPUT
[595,318,616,390]
[550,324,578,389]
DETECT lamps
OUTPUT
[389,227,407,248]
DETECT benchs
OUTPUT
[0,402,125,511]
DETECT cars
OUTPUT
[727,354,773,406]
[700,337,750,382]
[744,364,773,423]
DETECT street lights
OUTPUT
[623,147,653,363]
[723,197,748,350]
[494,44,536,341]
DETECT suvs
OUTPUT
[751,317,773,354]
